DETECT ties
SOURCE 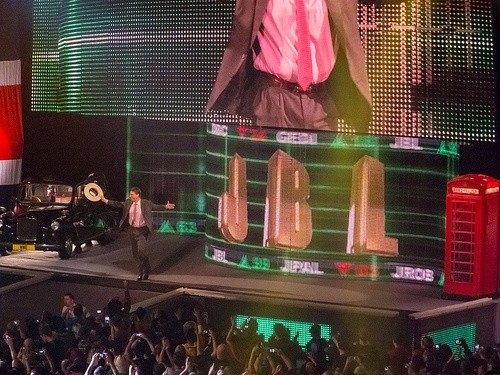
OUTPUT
[132,203,136,225]
[295,0,314,92]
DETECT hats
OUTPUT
[310,324,321,333]
[84,183,103,202]
[7,319,20,330]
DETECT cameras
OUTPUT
[96,309,102,314]
[104,315,110,323]
[39,349,44,354]
[135,333,142,336]
[247,317,250,324]
[435,344,439,351]
[269,348,276,353]
[98,354,105,359]
[455,339,462,345]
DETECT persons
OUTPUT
[0,293,499,375]
[101,186,176,282]
[204,1,374,131]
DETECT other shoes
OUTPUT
[138,273,143,280]
[142,273,149,280]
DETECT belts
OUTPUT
[252,68,330,94]
[129,225,147,229]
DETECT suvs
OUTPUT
[0,176,117,259]
